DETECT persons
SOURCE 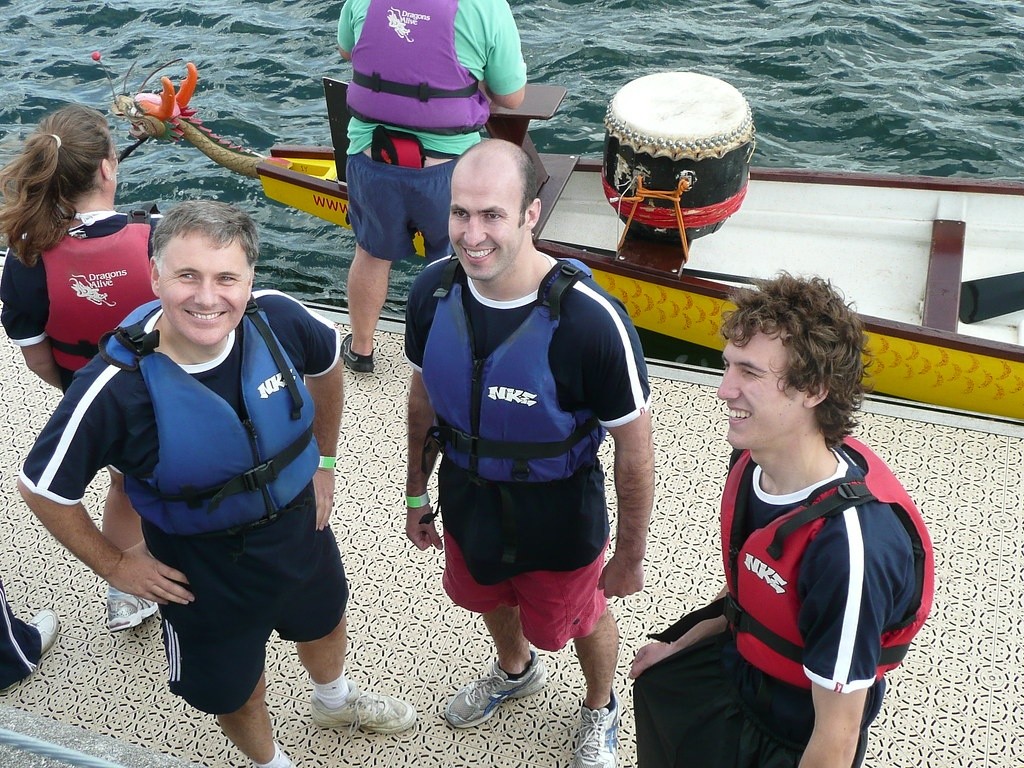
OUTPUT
[403,138,654,768]
[15,202,416,768]
[0,577,59,690]
[630,270,934,768]
[337,0,528,371]
[0,103,155,633]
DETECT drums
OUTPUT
[600,70,756,241]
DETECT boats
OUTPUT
[86,50,1024,424]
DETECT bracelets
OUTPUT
[319,456,336,468]
[407,492,430,508]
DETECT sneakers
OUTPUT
[107,591,159,632]
[1,607,58,690]
[311,680,417,737]
[341,334,374,374]
[444,651,548,729]
[574,688,619,768]
